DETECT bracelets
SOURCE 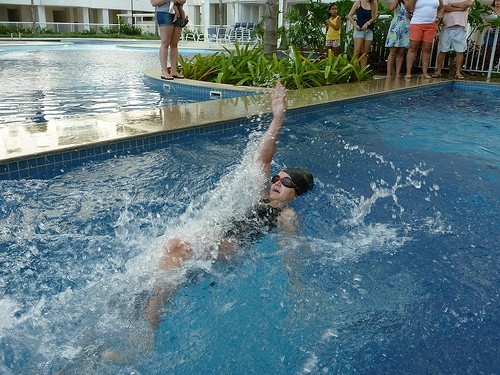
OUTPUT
[367,22,370,25]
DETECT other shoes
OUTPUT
[161,75,174,80]
[455,75,464,80]
[173,73,184,78]
[172,14,181,23]
[180,19,189,27]
[432,74,442,78]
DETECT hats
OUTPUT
[280,168,313,194]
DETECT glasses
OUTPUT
[271,174,297,189]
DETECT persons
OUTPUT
[255,80,316,232]
[324,0,500,79]
[150,0,188,80]
